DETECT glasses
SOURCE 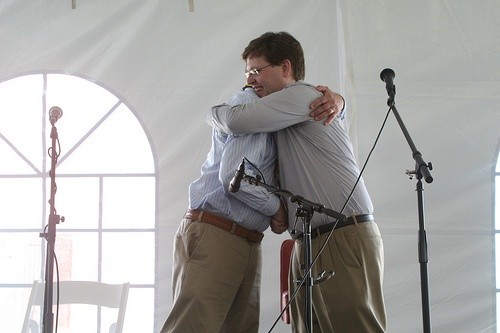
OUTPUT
[245,64,272,78]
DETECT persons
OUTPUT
[202,30,388,333]
[160,81,346,333]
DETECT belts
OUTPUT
[184,211,264,244]
[298,214,374,241]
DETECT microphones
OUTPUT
[229,159,244,193]
[380,68,395,101]
[48,106,63,123]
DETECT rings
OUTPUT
[328,107,336,117]
